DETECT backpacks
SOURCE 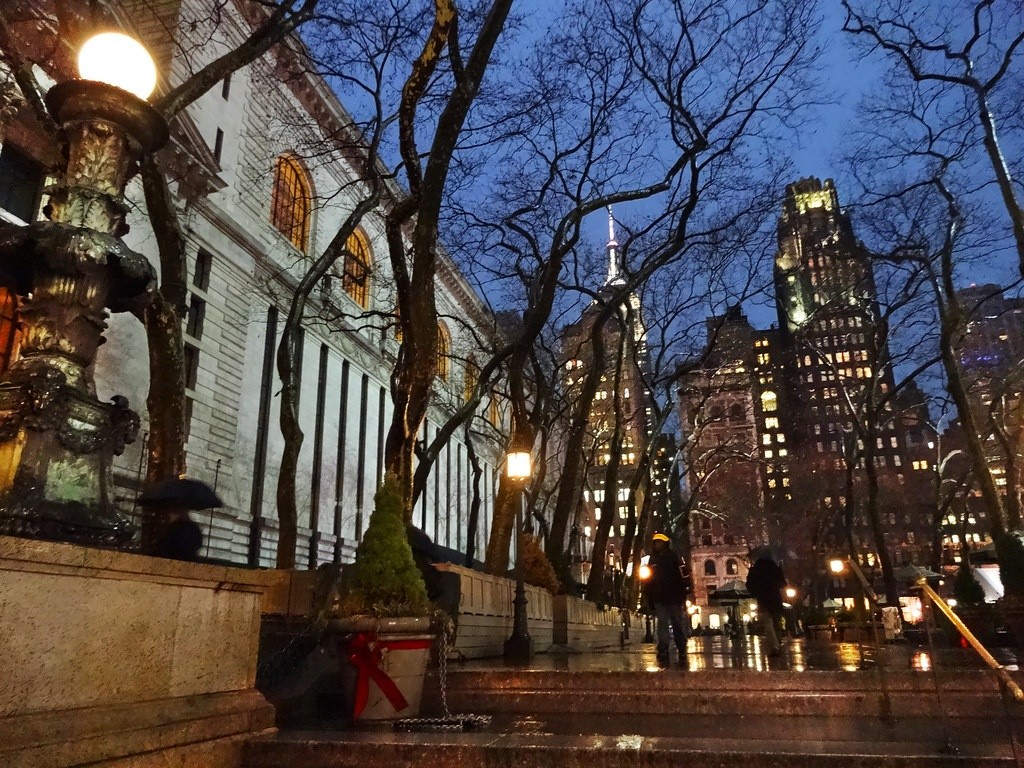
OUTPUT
[746,563,778,598]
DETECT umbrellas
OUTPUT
[814,598,843,610]
[718,598,744,617]
[708,579,752,622]
[896,561,945,583]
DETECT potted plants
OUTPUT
[317,464,434,729]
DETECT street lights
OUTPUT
[503,443,536,665]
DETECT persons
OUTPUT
[645,532,689,666]
[134,474,225,515]
[745,548,788,656]
[695,615,803,639]
[164,509,203,558]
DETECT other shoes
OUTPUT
[657,653,668,658]
[679,650,687,657]
